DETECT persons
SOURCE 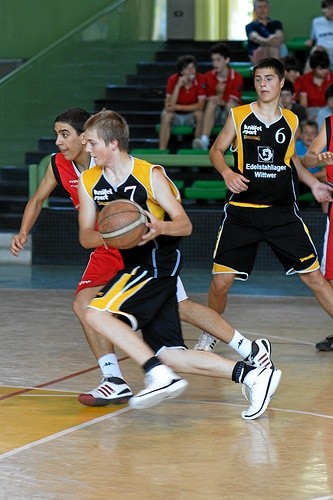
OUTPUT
[159,0,333,174]
[77,110,282,419]
[301,115,333,352]
[11,108,274,408]
[192,59,333,352]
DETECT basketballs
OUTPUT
[98,198,148,251]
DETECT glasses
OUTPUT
[254,77,279,83]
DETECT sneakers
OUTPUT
[315,336,333,351]
[79,378,133,405]
[241,367,282,420]
[244,338,273,367]
[128,365,190,409]
[193,331,221,351]
[192,139,208,151]
